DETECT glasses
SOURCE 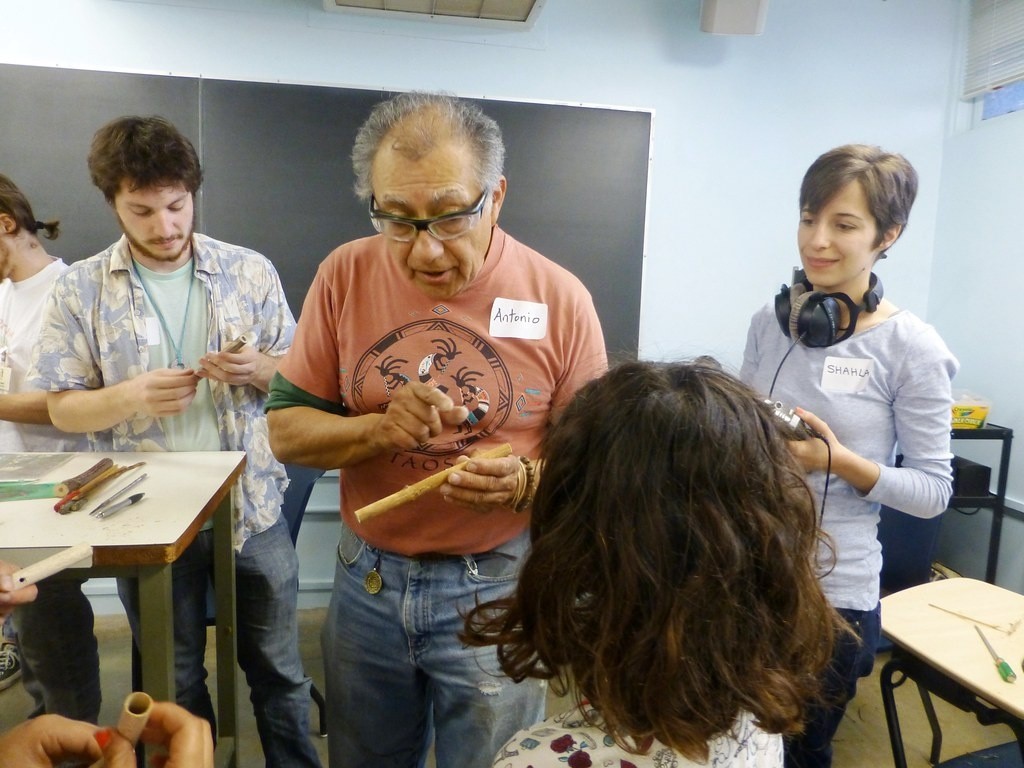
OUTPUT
[369,188,489,242]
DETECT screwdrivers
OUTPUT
[974,624,1017,683]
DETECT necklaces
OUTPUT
[124,242,202,373]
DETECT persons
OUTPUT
[738,143,958,768]
[0,114,323,767]
[452,353,864,768]
[265,90,607,768]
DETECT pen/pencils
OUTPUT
[95,492,146,519]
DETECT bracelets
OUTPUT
[517,453,532,517]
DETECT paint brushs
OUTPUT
[89,473,147,515]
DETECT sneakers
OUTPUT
[0,642,22,691]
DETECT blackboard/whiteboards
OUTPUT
[0,63,656,477]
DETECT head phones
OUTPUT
[775,268,884,349]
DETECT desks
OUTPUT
[879,577,1024,768]
[0,449,249,768]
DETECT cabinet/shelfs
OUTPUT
[896,423,1013,584]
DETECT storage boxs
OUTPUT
[952,390,991,428]
[951,456,992,497]
